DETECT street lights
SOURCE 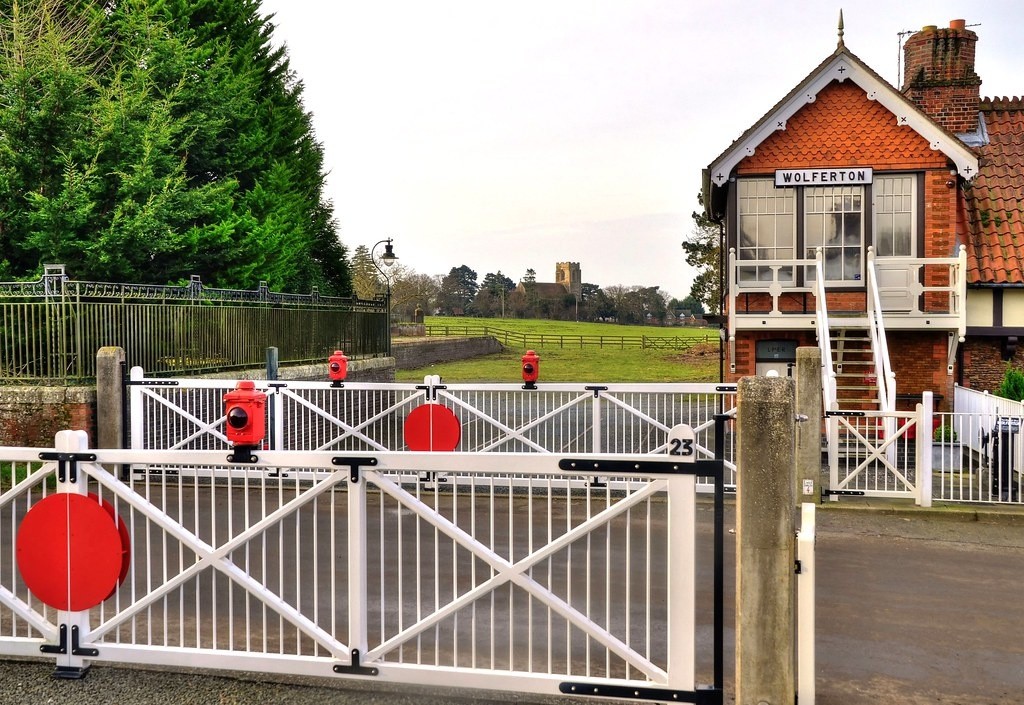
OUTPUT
[369,237,399,357]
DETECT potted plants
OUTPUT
[933,425,967,473]
